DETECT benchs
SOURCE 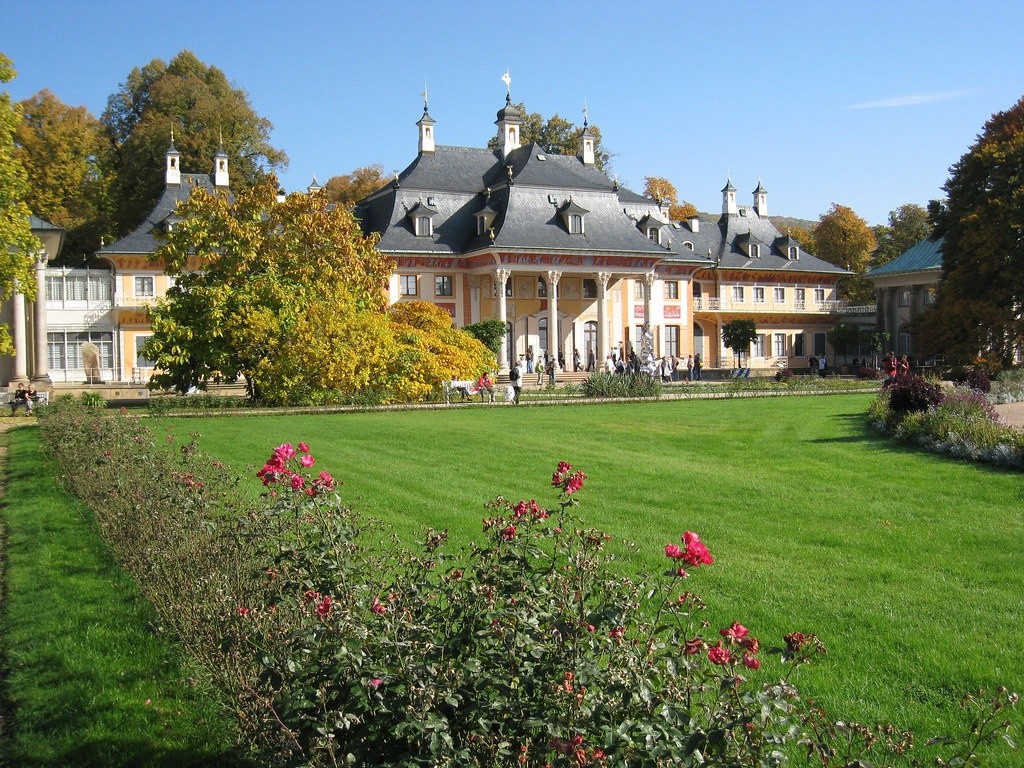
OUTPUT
[443,380,492,403]
[0,392,49,409]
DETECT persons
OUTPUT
[475,372,494,401]
[882,352,909,379]
[452,375,473,402]
[809,354,826,376]
[526,341,705,386]
[11,383,37,416]
[509,361,522,406]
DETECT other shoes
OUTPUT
[511,399,516,406]
[468,398,473,401]
[26,410,33,416]
[491,398,494,402]
[482,398,485,402]
[537,383,543,385]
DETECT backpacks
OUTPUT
[510,368,519,381]
